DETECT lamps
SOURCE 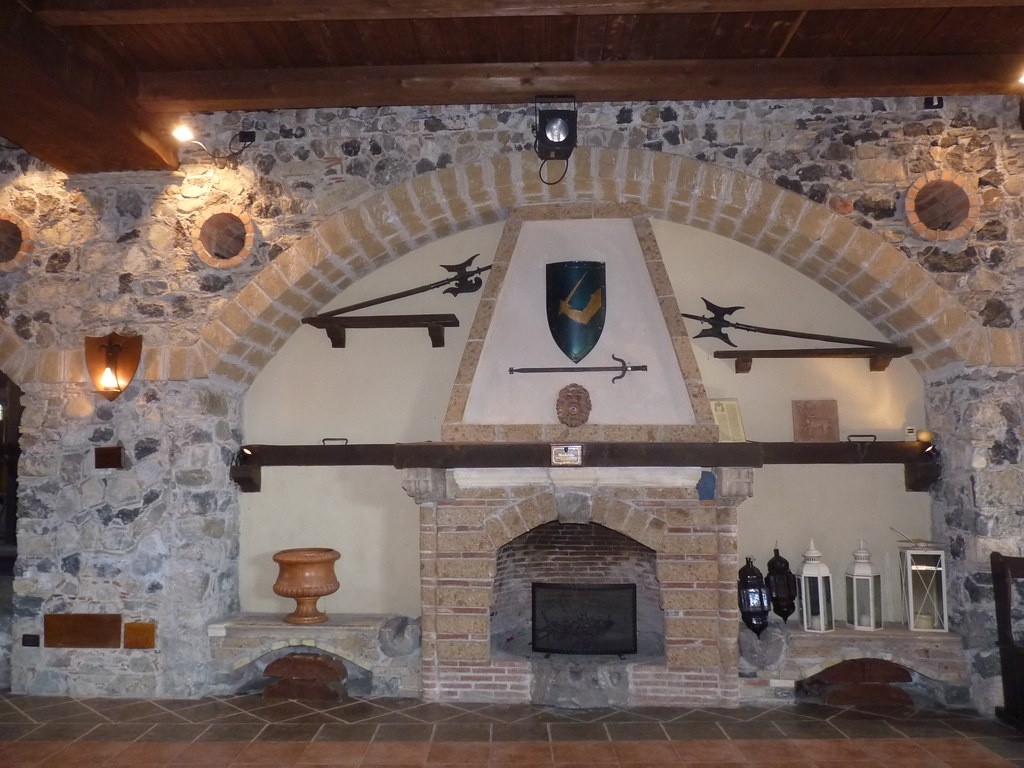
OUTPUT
[737,557,768,632]
[844,537,884,632]
[84,333,142,401]
[797,537,834,633]
[765,548,797,621]
[533,95,577,186]
[890,528,947,633]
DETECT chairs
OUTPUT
[989,551,1024,732]
